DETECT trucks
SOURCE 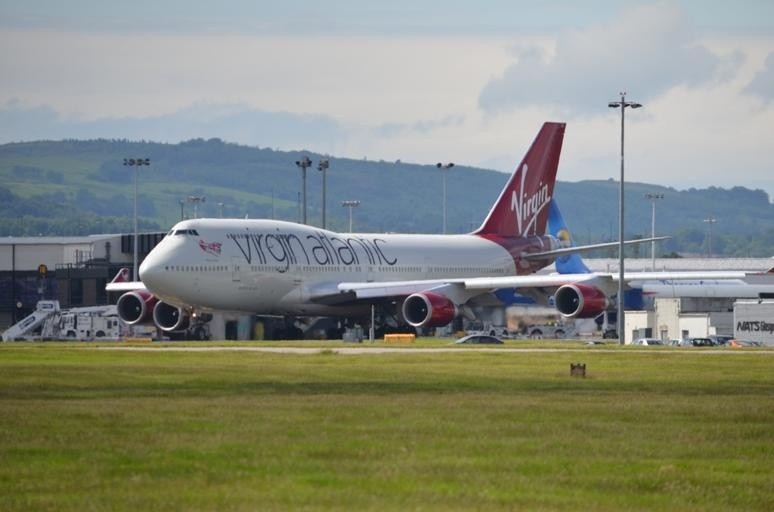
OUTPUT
[730,297,773,347]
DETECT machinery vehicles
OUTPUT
[0,300,159,341]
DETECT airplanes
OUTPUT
[102,113,675,345]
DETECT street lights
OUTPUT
[177,199,185,220]
[315,157,330,232]
[641,190,666,271]
[435,160,451,234]
[341,199,360,235]
[608,90,641,346]
[189,194,206,217]
[297,157,315,227]
[120,153,160,283]
[701,217,718,259]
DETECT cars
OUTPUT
[445,333,504,344]
[582,334,761,347]
